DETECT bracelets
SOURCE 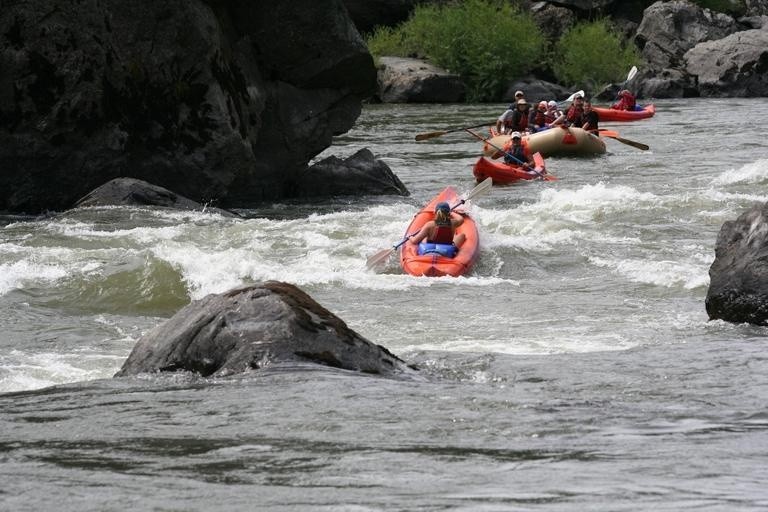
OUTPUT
[408,235,414,237]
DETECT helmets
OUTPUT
[539,101,547,110]
[548,99,557,110]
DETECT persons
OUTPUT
[490,131,535,171]
[607,89,637,111]
[493,90,600,139]
[405,202,466,259]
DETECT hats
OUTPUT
[436,203,451,212]
[514,90,523,97]
[510,131,521,140]
[574,93,582,100]
[517,98,527,106]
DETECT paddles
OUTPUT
[598,130,619,137]
[558,89,585,106]
[608,65,638,108]
[463,128,559,180]
[415,122,496,141]
[366,177,492,268]
[610,136,650,150]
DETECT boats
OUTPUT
[400,184,480,277]
[592,104,655,122]
[473,126,607,184]
